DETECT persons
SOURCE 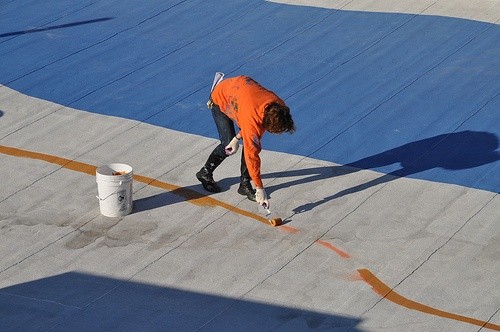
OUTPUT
[196,76,296,209]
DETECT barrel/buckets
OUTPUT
[96,164,133,217]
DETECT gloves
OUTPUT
[224,136,239,157]
[255,186,271,210]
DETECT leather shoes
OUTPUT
[196,168,222,193]
[237,184,256,202]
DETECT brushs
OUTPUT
[263,203,282,227]
